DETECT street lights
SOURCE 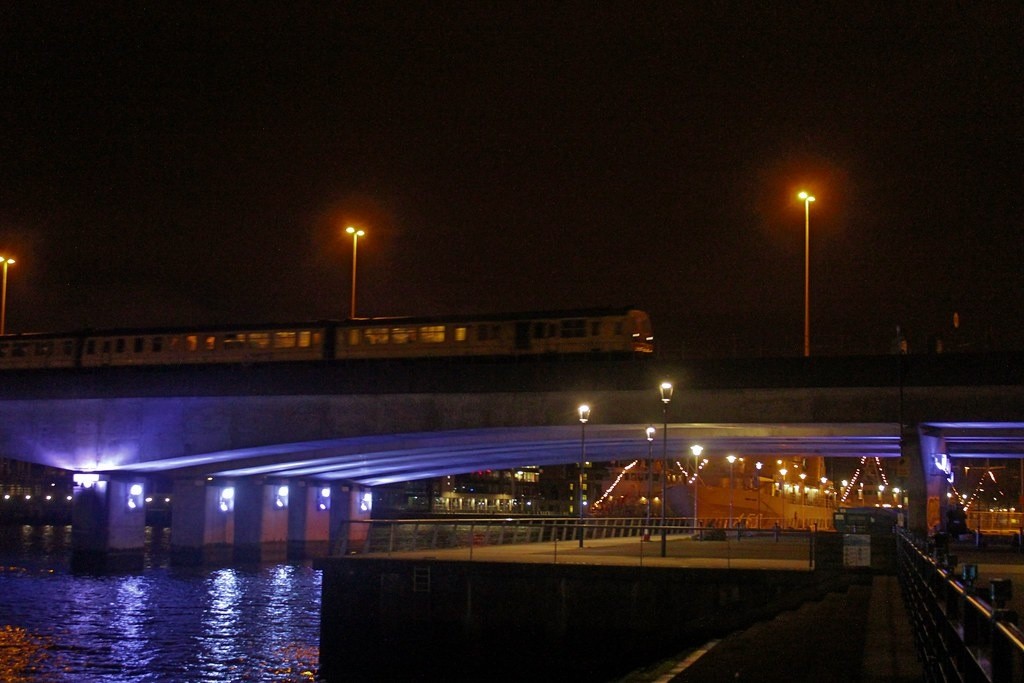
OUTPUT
[726,454,737,529]
[799,473,808,528]
[779,468,789,529]
[690,444,703,538]
[645,426,655,542]
[0,257,17,336]
[820,476,828,530]
[658,381,675,559]
[577,404,586,550]
[345,227,366,320]
[798,190,814,357]
[755,462,764,532]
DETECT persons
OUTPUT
[773,522,781,542]
